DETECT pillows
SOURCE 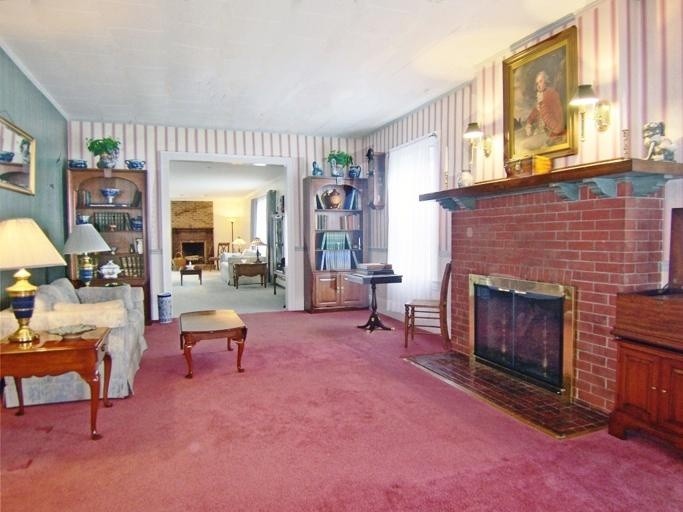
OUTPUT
[37,278,133,317]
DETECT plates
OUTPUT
[48,324,98,339]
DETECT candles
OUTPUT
[444,147,448,172]
[622,97,628,130]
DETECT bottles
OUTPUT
[98,261,123,278]
[349,165,360,178]
[67,160,87,168]
[326,189,342,207]
[125,159,144,170]
[458,170,471,187]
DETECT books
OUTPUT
[76,190,145,277]
[317,189,393,275]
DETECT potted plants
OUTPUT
[327,149,353,176]
[85,135,121,168]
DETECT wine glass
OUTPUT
[100,188,120,203]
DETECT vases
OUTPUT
[157,292,172,324]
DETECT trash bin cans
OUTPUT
[158,293,171,324]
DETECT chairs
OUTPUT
[404,262,451,349]
[209,242,230,272]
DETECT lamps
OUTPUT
[231,237,247,252]
[249,237,266,263]
[569,84,610,142]
[463,122,492,165]
[0,218,68,342]
[63,224,111,287]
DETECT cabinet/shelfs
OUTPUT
[608,339,683,461]
[303,176,370,313]
[66,168,152,327]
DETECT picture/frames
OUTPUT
[0,115,36,195]
[502,25,578,168]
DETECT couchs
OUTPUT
[220,249,267,286]
[0,287,149,408]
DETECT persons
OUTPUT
[519,70,567,150]
